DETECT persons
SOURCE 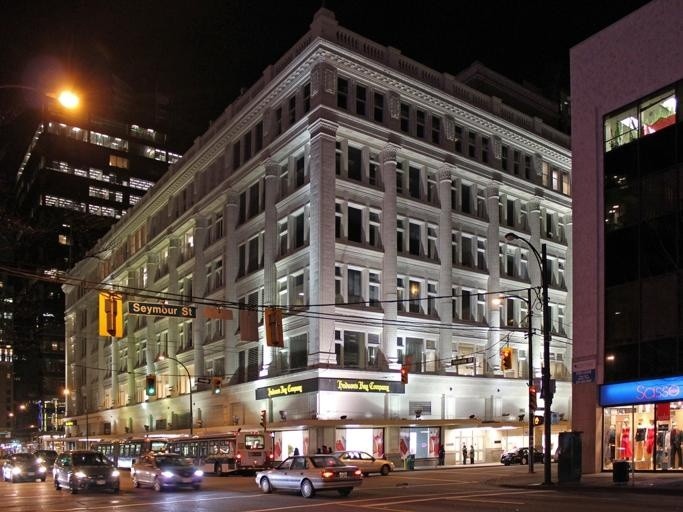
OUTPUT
[438,444,444,465]
[463,445,467,464]
[317,446,332,454]
[294,448,299,455]
[607,419,683,470]
[469,445,474,464]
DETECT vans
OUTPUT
[52,450,121,495]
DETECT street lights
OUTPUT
[505,232,554,487]
[63,389,88,451]
[158,354,192,434]
[491,289,535,474]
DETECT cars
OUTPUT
[327,450,395,476]
[542,445,559,463]
[29,450,58,474]
[129,452,203,491]
[255,453,363,499]
[1,452,46,483]
[500,447,545,466]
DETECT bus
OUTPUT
[161,430,272,476]
[89,437,168,471]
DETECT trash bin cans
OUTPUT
[404,454,415,471]
[613,462,630,486]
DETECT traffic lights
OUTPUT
[532,416,543,425]
[145,375,156,396]
[400,367,407,384]
[211,376,221,395]
[500,348,512,370]
[529,385,536,411]
[260,410,265,429]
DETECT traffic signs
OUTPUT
[126,301,197,319]
[450,356,473,364]
